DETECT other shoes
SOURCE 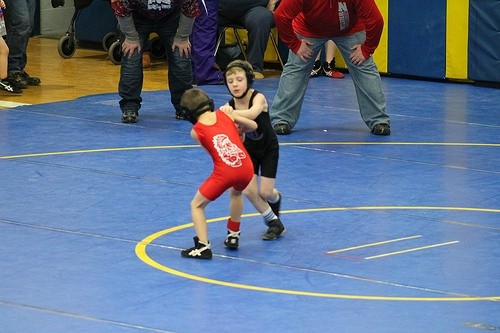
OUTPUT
[309,60,322,78]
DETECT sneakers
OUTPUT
[268,192,281,216]
[322,57,345,78]
[372,124,391,135]
[181,236,212,259]
[9,74,29,89]
[262,216,286,240]
[122,109,138,122]
[175,112,185,119]
[0,77,22,93]
[224,227,241,248]
[252,71,264,78]
[21,70,41,85]
[272,123,291,135]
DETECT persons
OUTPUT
[220,60,287,240]
[0,0,41,95]
[310,40,345,78]
[143,0,289,86]
[111,0,200,124]
[180,89,258,260]
[269,0,391,136]
